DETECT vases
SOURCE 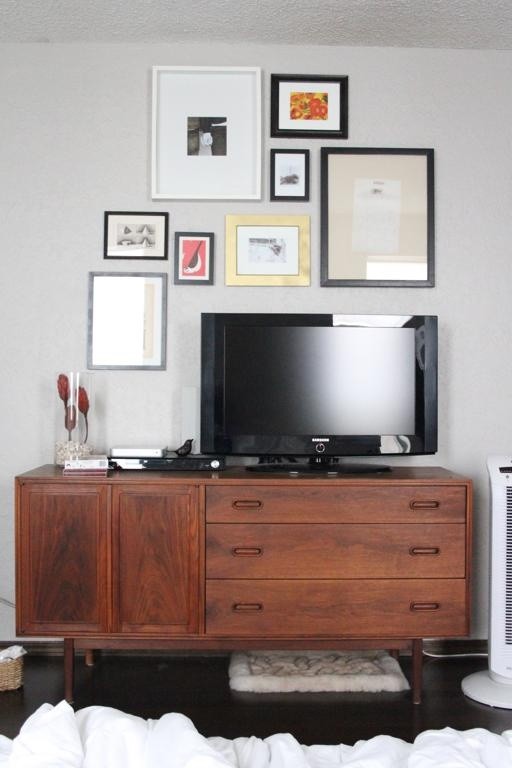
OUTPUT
[54,367,94,467]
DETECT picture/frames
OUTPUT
[320,146,435,288]
[270,148,310,202]
[151,64,262,199]
[103,210,169,260]
[224,213,310,287]
[270,73,349,141]
[87,270,168,371]
[174,231,214,285]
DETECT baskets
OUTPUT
[0,656,24,692]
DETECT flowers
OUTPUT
[58,373,89,442]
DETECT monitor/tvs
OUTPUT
[200,312,438,474]
